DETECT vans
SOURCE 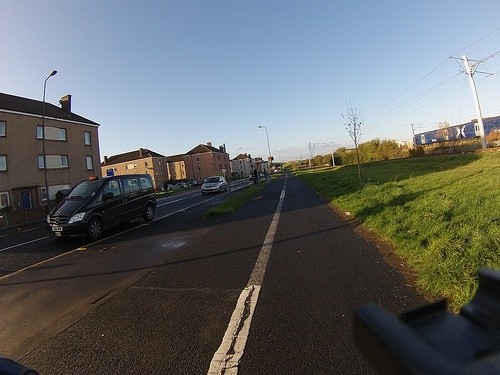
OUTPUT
[45,173,158,244]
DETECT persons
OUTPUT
[164,182,168,194]
[264,169,268,180]
[253,169,258,184]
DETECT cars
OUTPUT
[160,178,198,192]
[200,175,227,195]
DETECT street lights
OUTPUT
[41,70,57,212]
[258,125,272,163]
